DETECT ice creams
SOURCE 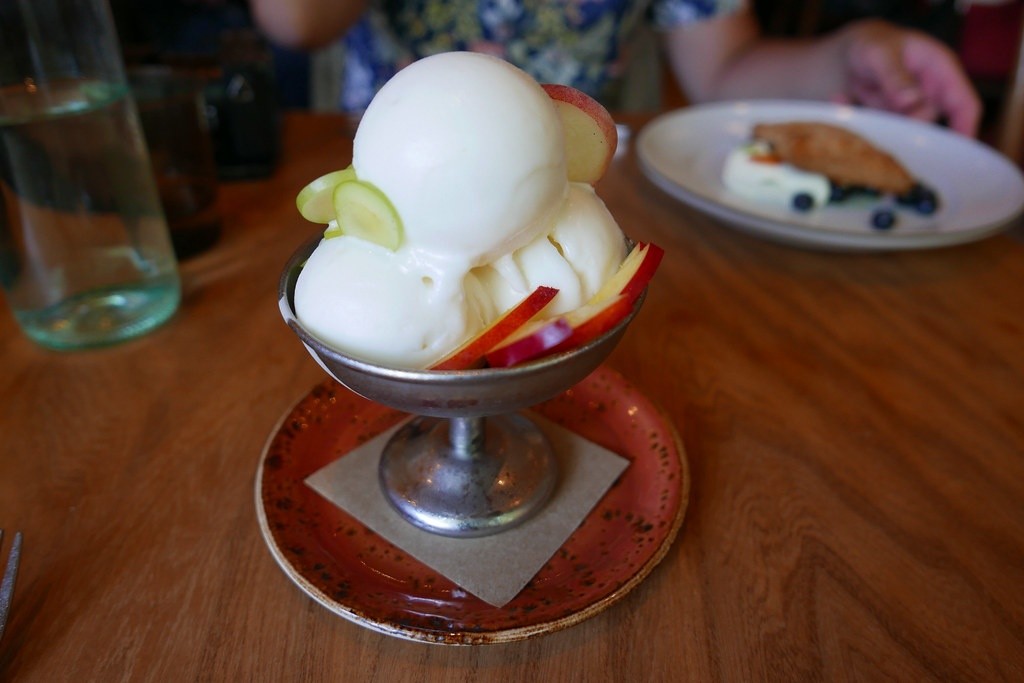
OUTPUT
[293,51,628,371]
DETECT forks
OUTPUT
[0,529,23,643]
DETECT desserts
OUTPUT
[720,119,919,213]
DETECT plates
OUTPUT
[253,376,691,648]
[633,98,1023,253]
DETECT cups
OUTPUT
[127,69,222,262]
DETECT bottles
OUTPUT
[0,0,182,350]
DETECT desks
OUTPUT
[0,114,1024,683]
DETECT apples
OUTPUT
[429,242,664,369]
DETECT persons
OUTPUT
[250,1,982,139]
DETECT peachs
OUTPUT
[538,83,618,184]
